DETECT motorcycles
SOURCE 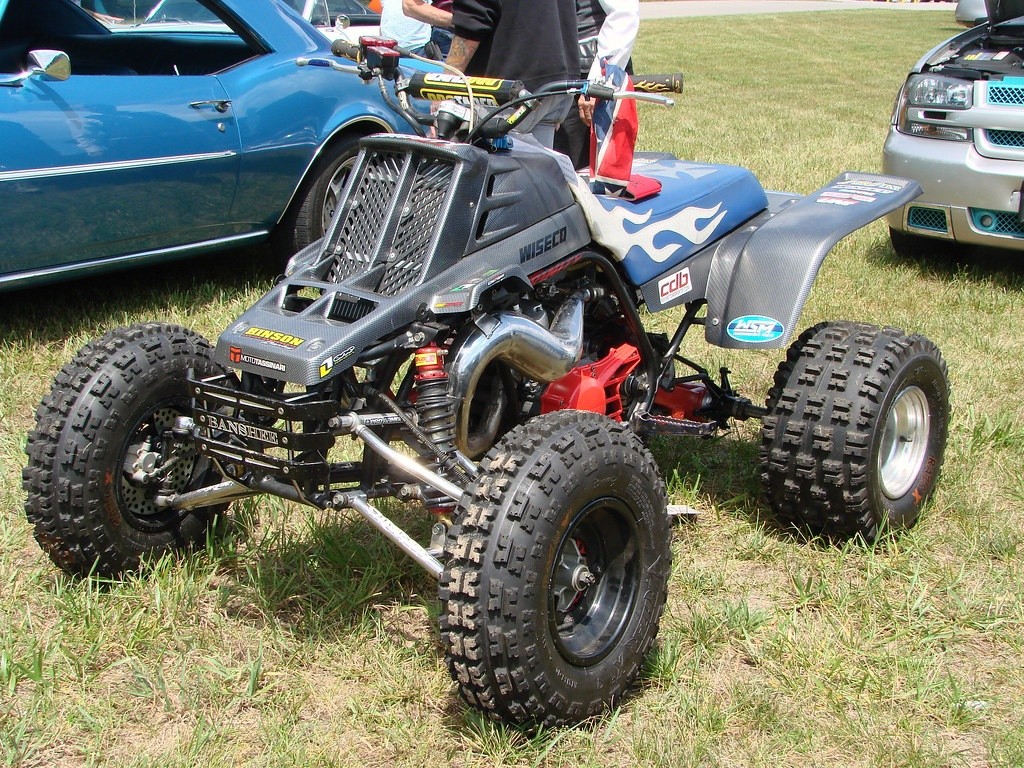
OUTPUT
[20,35,950,734]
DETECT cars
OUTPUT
[0,0,460,289]
[875,0,1024,262]
[75,0,384,47]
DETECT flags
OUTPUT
[590,59,639,197]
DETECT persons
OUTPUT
[379,0,641,172]
